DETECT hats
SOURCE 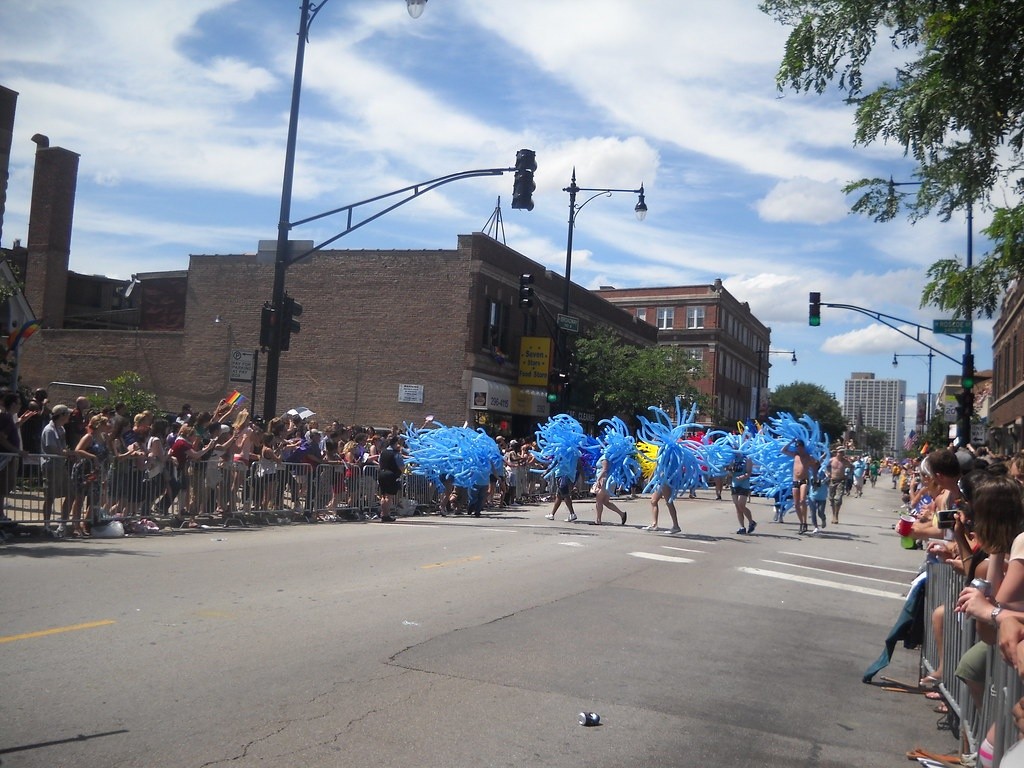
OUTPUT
[510,440,518,444]
[51,404,74,414]
[495,436,505,440]
[837,446,845,453]
[903,463,909,468]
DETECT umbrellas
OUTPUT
[6,320,41,348]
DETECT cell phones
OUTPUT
[937,510,957,522]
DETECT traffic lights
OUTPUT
[547,392,557,403]
[518,273,534,308]
[260,305,279,347]
[809,292,820,326]
[512,149,538,212]
[282,296,302,351]
[963,354,974,388]
[954,393,973,417]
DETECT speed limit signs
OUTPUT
[944,395,958,422]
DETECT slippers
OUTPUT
[935,705,949,712]
[590,522,601,525]
[622,512,626,525]
[920,677,943,685]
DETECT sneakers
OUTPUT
[664,527,680,534]
[382,516,396,522]
[747,521,757,533]
[642,525,659,530]
[545,513,554,520]
[564,514,576,521]
[737,528,746,533]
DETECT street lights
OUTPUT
[756,347,797,421]
[882,175,973,447]
[892,349,935,422]
[264,0,427,418]
[559,183,647,413]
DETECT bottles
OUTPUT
[578,711,600,726]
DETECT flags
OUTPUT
[904,429,919,451]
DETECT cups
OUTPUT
[898,514,916,536]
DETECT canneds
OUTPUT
[968,577,992,598]
[577,712,601,725]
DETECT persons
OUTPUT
[852,455,867,498]
[642,434,682,534]
[781,438,816,535]
[823,446,851,525]
[40,405,72,536]
[590,439,627,525]
[891,462,897,489]
[726,446,757,534]
[0,392,29,525]
[377,437,405,522]
[545,439,579,521]
[869,461,879,487]
[466,445,496,518]
[0,378,1023,768]
[261,432,285,516]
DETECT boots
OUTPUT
[799,524,802,534]
[803,524,807,532]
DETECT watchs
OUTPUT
[991,606,1003,630]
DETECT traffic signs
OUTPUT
[970,425,984,444]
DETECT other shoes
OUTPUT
[821,519,826,528]
[813,528,819,533]
[36,521,94,539]
